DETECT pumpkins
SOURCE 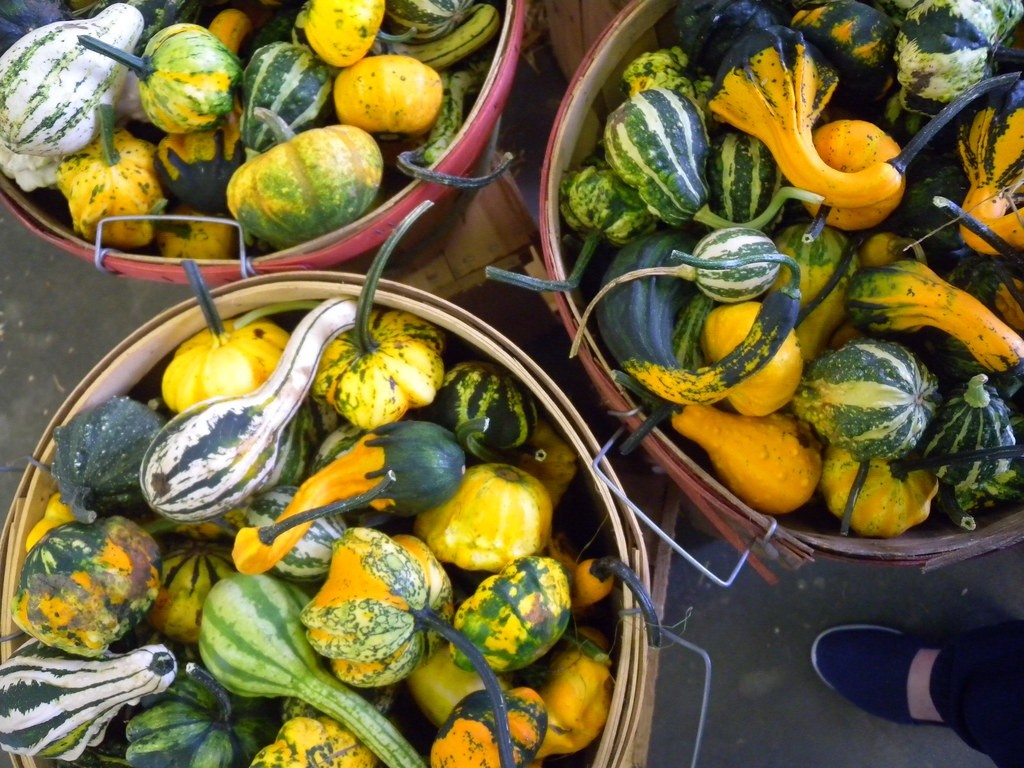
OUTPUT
[1,0,1024,768]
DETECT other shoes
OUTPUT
[808,623,947,729]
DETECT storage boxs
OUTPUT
[365,151,542,335]
[0,0,526,286]
[1,270,711,767]
[544,0,627,85]
[538,0,1024,591]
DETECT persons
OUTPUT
[809,613,1022,768]
